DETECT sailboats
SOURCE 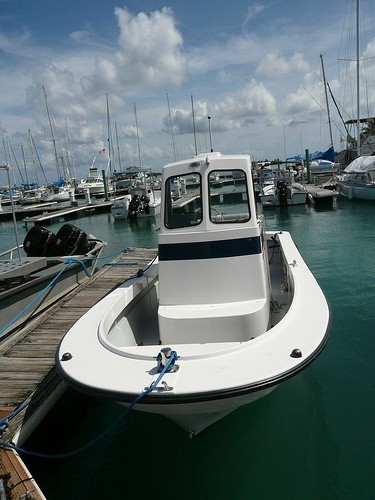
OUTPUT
[0,128,108,339]
[0,0,375,229]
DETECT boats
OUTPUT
[54,112,335,441]
